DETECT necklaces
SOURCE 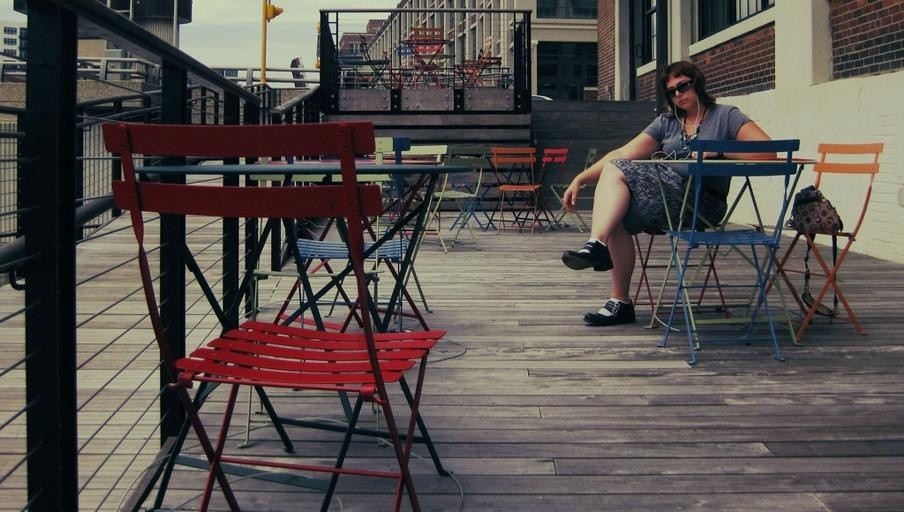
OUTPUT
[679,108,710,145]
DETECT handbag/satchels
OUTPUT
[792,185,842,232]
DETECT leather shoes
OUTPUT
[584,297,636,326]
[562,241,615,272]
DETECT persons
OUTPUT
[561,60,777,331]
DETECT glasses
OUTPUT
[667,81,692,97]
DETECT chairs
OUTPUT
[748,137,884,342]
[653,130,803,368]
[626,227,733,321]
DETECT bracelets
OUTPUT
[718,151,723,157]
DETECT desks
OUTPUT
[625,153,822,349]
